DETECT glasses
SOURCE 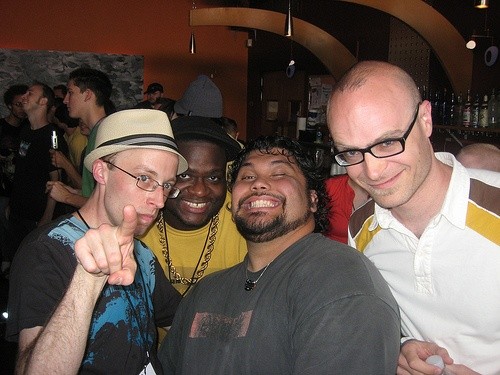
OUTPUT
[9,102,22,107]
[102,160,181,199]
[331,101,423,168]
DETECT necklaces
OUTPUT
[245,253,275,291]
[156,207,220,294]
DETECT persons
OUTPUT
[0,79,70,326]
[323,174,372,244]
[52,84,66,99]
[455,143,500,172]
[157,130,402,375]
[169,75,245,162]
[223,118,240,141]
[0,85,31,261]
[134,116,250,354]
[49,99,117,191]
[45,67,111,208]
[325,60,500,375]
[132,83,177,120]
[6,108,189,375]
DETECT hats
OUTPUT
[174,75,223,117]
[55,104,68,123]
[170,116,242,162]
[144,83,163,95]
[83,108,189,175]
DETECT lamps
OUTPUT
[465,36,476,50]
[285,0,294,37]
[189,0,196,55]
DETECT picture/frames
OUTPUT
[0,48,144,127]
